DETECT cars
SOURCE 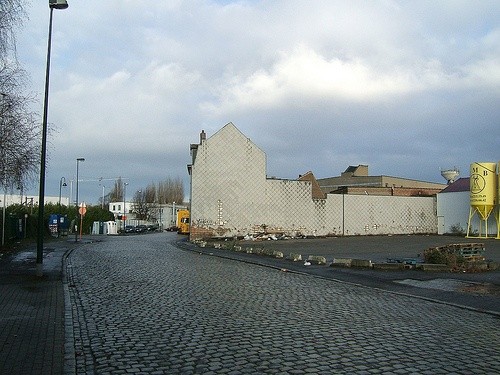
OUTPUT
[166,226,179,232]
[135,224,161,233]
[126,225,136,233]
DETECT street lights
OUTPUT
[123,182,128,230]
[75,157,86,207]
[36,1,69,264]
[17,184,23,205]
[58,176,68,204]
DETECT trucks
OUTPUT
[178,210,190,234]
